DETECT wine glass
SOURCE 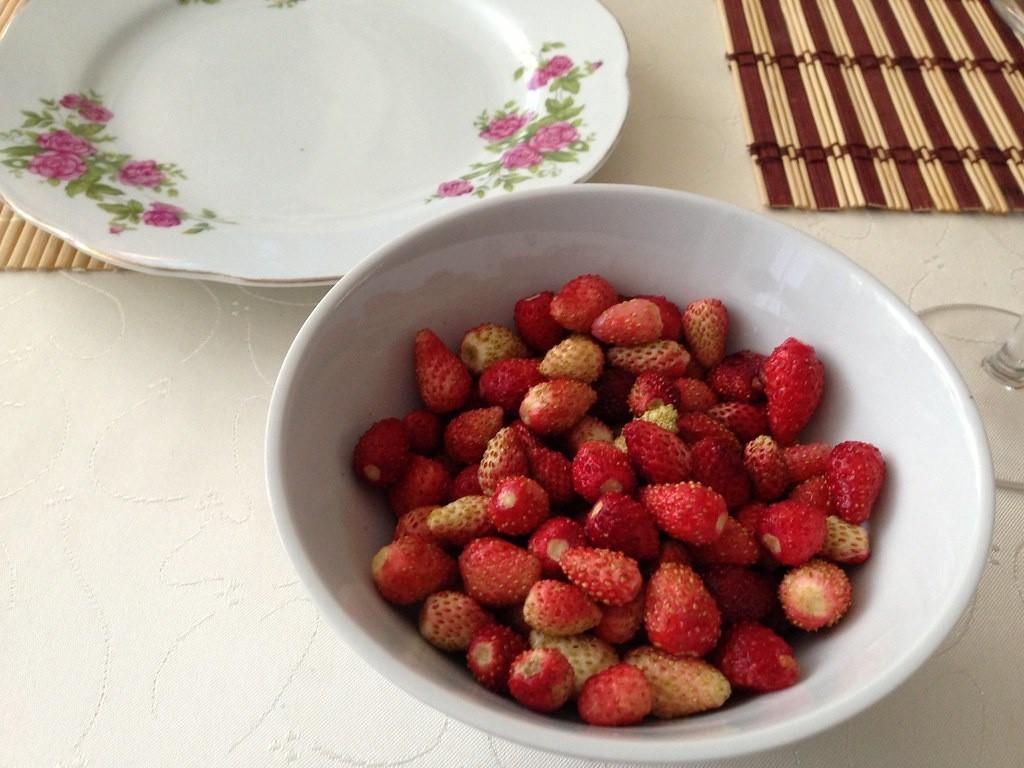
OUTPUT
[915,303,1024,491]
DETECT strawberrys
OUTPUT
[354,278,886,724]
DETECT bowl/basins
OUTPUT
[263,181,995,765]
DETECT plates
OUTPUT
[0,0,630,289]
